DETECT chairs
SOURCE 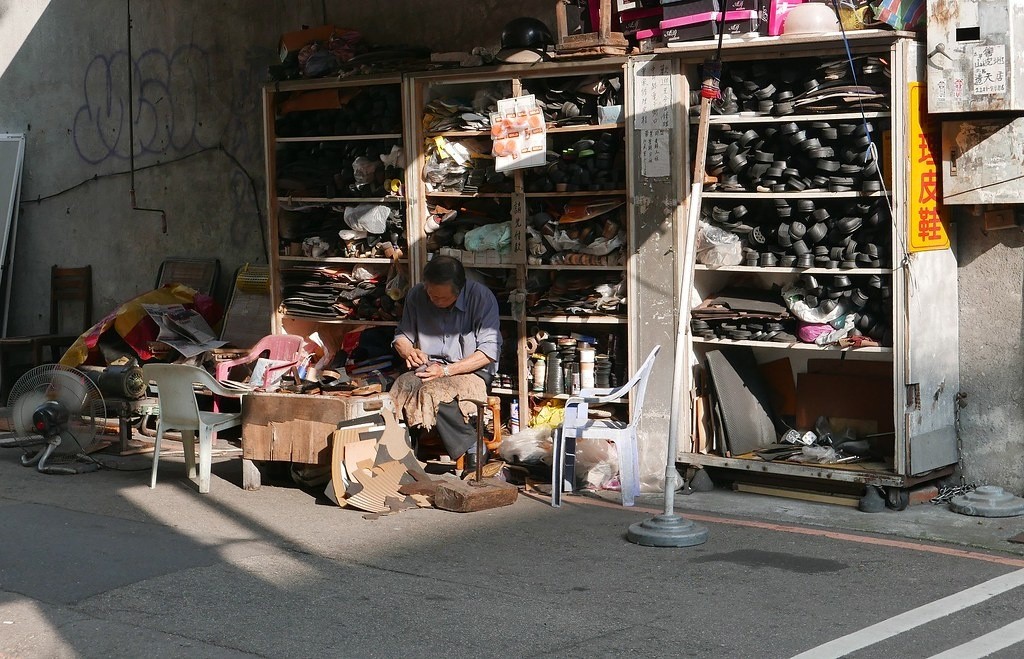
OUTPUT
[154,255,219,310]
[216,334,303,395]
[140,363,253,495]
[552,343,665,506]
[0,263,94,364]
[219,266,273,354]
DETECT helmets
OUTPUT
[501,17,554,50]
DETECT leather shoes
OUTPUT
[461,446,490,480]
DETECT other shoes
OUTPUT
[274,52,892,397]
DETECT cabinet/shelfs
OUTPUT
[259,24,955,507]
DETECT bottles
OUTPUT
[511,399,519,434]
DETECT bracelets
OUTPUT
[442,364,450,378]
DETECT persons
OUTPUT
[391,256,503,478]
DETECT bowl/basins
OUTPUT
[784,2,838,34]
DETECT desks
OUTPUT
[196,390,394,498]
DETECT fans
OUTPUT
[6,364,108,476]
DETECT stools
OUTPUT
[548,0,630,54]
[459,396,501,470]
